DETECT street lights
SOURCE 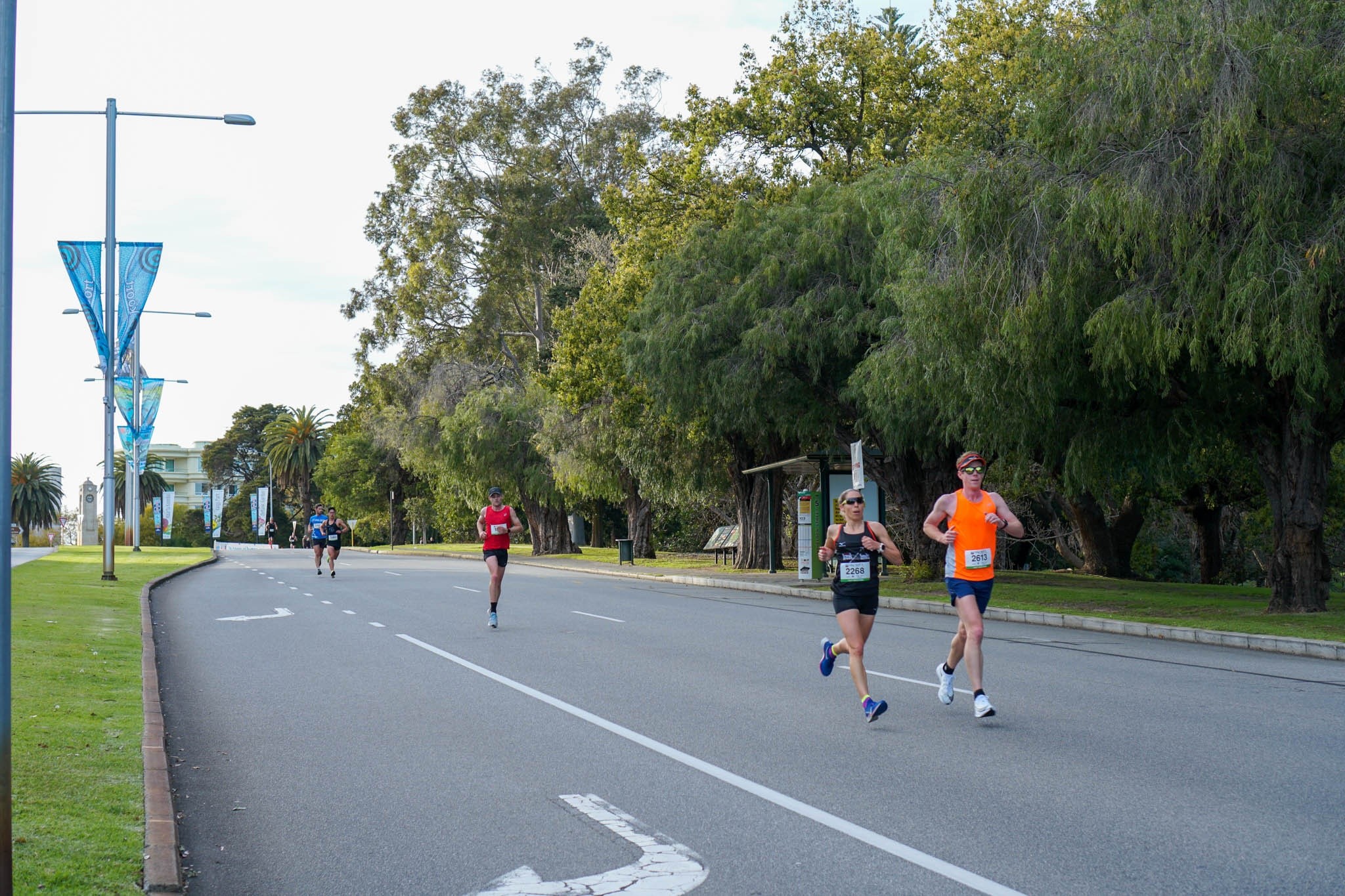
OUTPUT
[14,98,256,582]
[62,307,211,551]
[252,452,273,518]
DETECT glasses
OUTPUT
[961,465,984,475]
[841,497,864,505]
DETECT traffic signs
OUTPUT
[11,527,24,535]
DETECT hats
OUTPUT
[488,487,501,497]
[957,454,986,471]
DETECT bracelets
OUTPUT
[340,530,343,533]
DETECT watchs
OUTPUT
[508,529,511,533]
[999,519,1008,530]
[877,543,885,553]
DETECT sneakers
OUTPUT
[818,637,836,677]
[974,694,996,718]
[935,662,956,705]
[487,608,498,629]
[864,698,888,723]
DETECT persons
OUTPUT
[476,487,523,628]
[289,532,298,548]
[301,535,311,549]
[266,516,278,549]
[308,503,328,575]
[320,507,348,578]
[921,451,1024,718]
[818,489,900,725]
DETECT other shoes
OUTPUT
[331,570,335,579]
[326,555,330,563]
[317,569,322,575]
